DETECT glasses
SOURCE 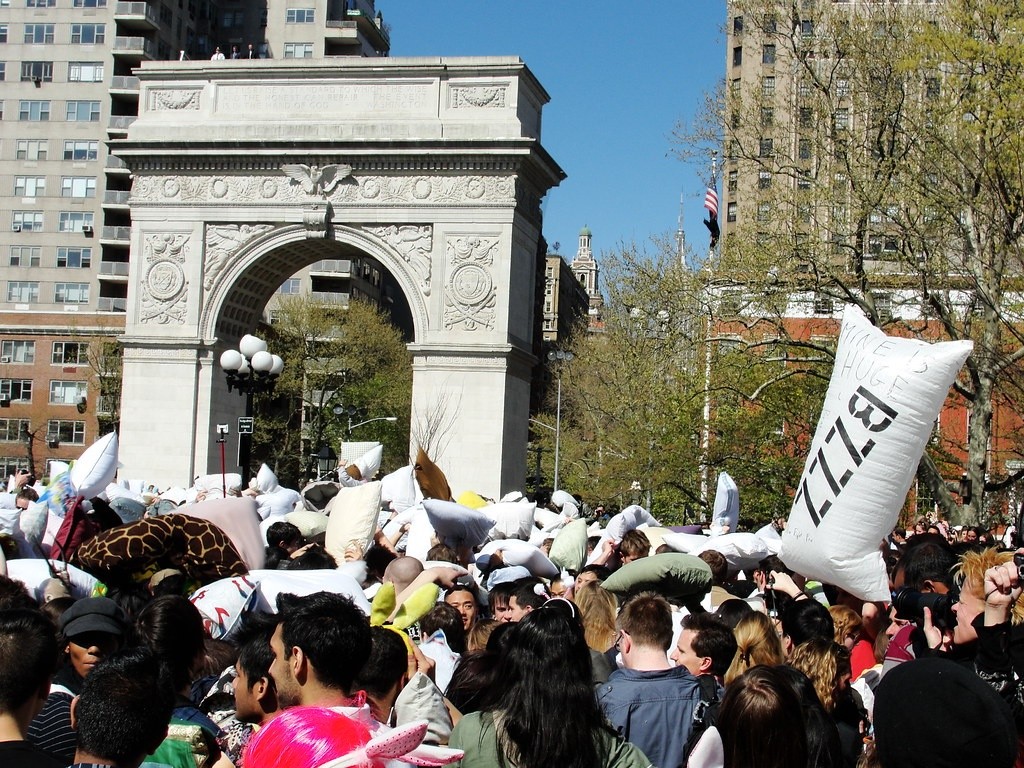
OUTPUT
[15,505,27,511]
[614,631,630,652]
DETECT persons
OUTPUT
[0,459,1024,768]
[439,608,650,768]
[269,592,376,726]
[65,657,172,768]
[210,44,261,60]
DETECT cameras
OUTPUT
[18,469,36,487]
[1013,552,1024,591]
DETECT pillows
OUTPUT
[0,302,975,641]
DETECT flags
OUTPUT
[704,173,719,219]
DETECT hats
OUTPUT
[60,597,132,638]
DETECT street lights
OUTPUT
[219,330,284,495]
[627,305,673,515]
[545,349,575,494]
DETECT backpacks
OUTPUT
[681,674,732,768]
